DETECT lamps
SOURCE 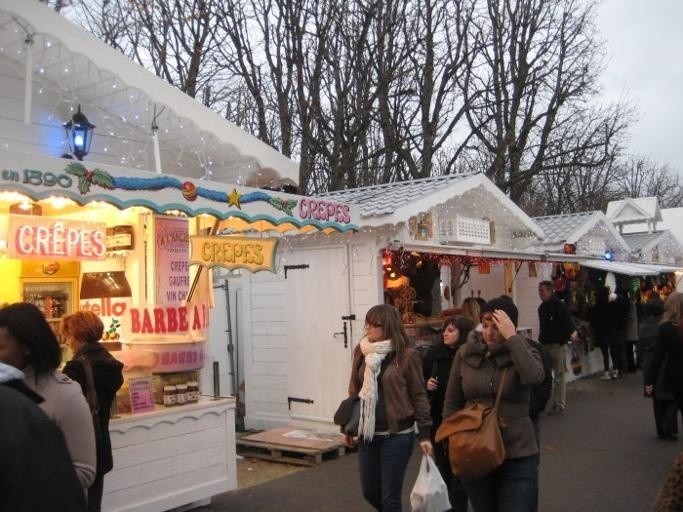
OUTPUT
[62,103,96,160]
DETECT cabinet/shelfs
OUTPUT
[401,316,459,347]
[22,276,76,369]
[100,396,236,512]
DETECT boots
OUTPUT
[600,370,618,380]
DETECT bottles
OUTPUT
[164,381,200,406]
[23,290,69,346]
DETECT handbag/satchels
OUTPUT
[334,396,360,436]
[92,418,113,476]
[434,403,506,475]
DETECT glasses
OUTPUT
[367,321,382,328]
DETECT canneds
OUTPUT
[176,384,188,404]
[163,386,177,405]
[186,381,199,403]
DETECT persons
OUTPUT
[420,314,479,509]
[462,275,683,444]
[440,292,548,510]
[0,300,97,498]
[0,360,88,509]
[342,304,436,511]
[59,307,125,511]
[524,337,554,470]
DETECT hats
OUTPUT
[480,295,518,328]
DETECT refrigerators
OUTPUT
[2,258,80,374]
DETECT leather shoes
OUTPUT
[548,406,561,415]
